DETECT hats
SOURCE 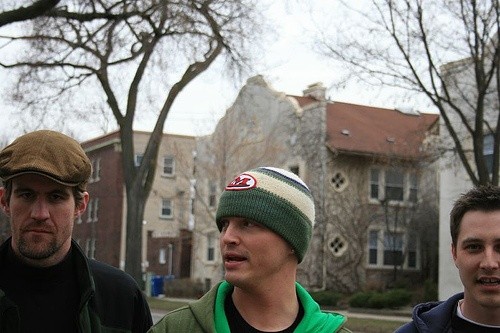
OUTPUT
[0,130,93,192]
[216,166,316,264]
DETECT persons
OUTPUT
[393,185,500,333]
[145,166,354,333]
[0,129,153,333]
